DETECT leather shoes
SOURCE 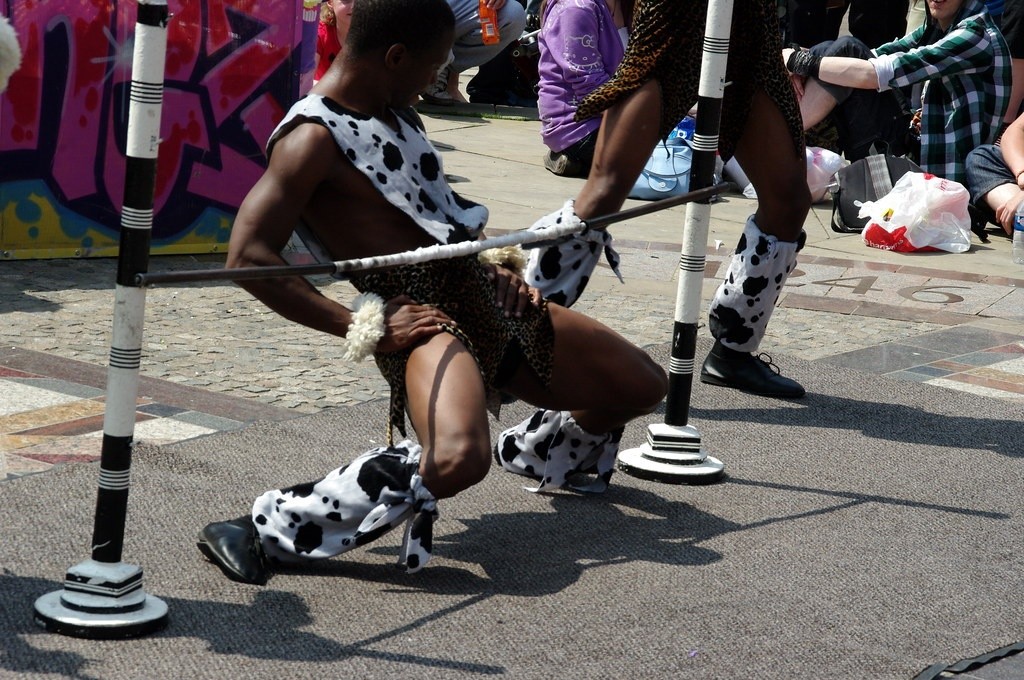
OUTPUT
[493,441,597,475]
[701,342,806,399]
[197,520,264,582]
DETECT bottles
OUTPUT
[1012,199,1024,264]
[479,0,500,46]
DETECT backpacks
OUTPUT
[828,139,927,232]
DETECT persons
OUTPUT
[421,0,759,199]
[777,0,1024,235]
[509,0,812,400]
[196,0,670,586]
[312,0,353,88]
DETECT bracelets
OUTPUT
[342,292,386,365]
[1016,168,1024,179]
[788,42,800,52]
[478,246,528,279]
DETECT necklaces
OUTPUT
[605,0,616,17]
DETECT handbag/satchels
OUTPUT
[854,171,973,254]
[466,40,539,109]
[626,138,693,198]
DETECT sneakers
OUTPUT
[542,150,571,174]
[420,67,454,106]
